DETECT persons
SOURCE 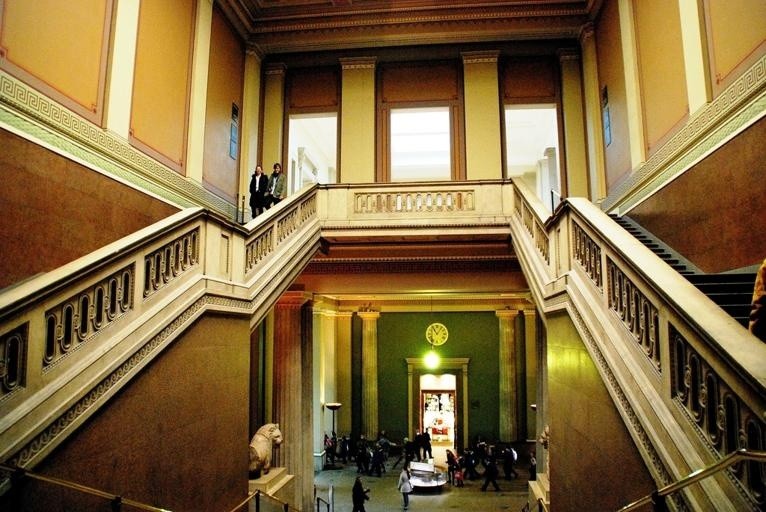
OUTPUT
[352,476,370,512]
[446,441,520,492]
[250,165,268,219]
[398,466,413,511]
[527,452,536,481]
[324,428,433,478]
[264,162,287,210]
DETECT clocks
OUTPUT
[426,322,448,348]
